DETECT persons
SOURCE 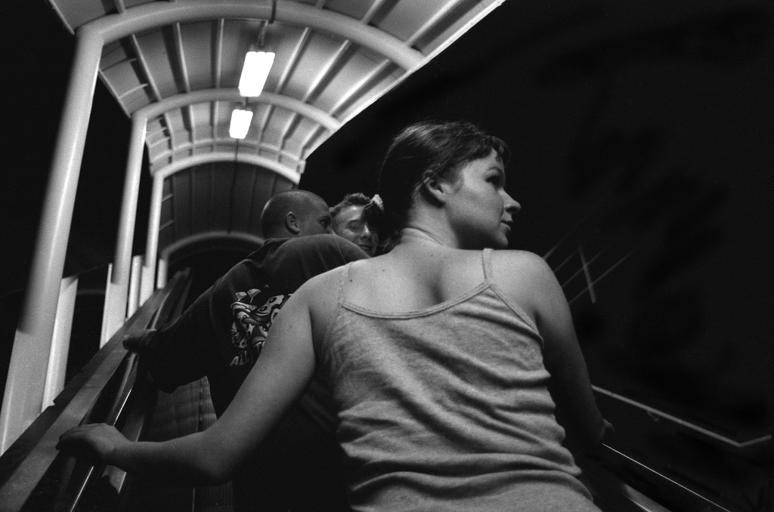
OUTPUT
[54,120,632,512]
[123,189,371,512]
[328,192,379,256]
